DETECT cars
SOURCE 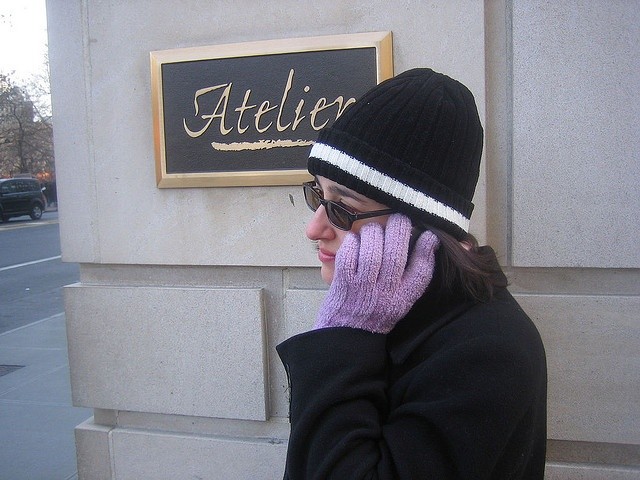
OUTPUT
[0,178,46,223]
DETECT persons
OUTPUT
[275,67,548,480]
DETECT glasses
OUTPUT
[302,181,398,231]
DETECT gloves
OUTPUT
[311,213,441,334]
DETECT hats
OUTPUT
[307,68,484,240]
